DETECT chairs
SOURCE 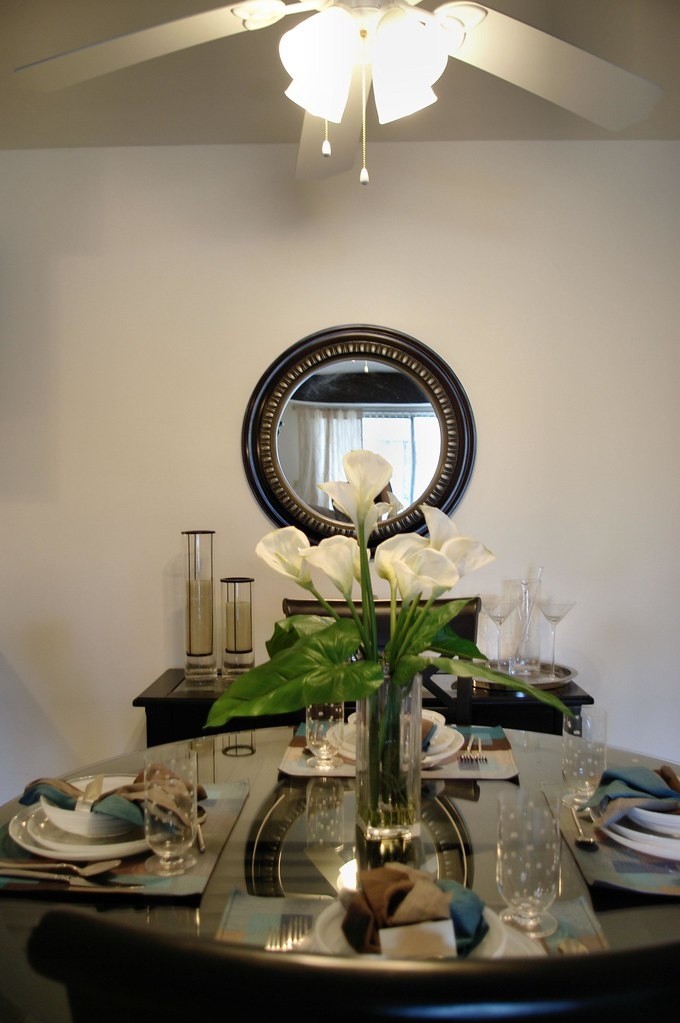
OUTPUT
[29,911,680,1023]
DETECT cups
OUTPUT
[502,578,541,677]
[145,904,201,938]
[305,776,345,853]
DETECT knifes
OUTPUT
[0,870,146,888]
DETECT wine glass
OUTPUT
[306,701,345,769]
[482,594,523,678]
[560,708,608,808]
[495,792,561,938]
[142,746,197,875]
[534,601,574,681]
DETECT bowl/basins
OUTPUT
[349,710,445,743]
[626,807,680,837]
[39,774,143,838]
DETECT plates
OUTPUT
[26,807,146,852]
[589,809,680,860]
[599,809,680,849]
[8,803,151,861]
[327,726,465,768]
[336,726,455,755]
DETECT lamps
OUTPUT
[230,0,488,185]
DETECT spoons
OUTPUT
[303,720,321,754]
[571,808,598,852]
[0,859,121,877]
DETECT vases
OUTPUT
[354,673,422,839]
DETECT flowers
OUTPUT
[201,448,580,728]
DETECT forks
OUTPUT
[475,737,488,762]
[460,733,474,760]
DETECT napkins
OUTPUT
[340,863,490,958]
[17,763,208,840]
[578,767,680,829]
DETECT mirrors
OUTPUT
[239,324,478,563]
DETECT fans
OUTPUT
[7,0,673,184]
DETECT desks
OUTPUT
[131,669,594,748]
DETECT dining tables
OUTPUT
[0,727,680,956]
